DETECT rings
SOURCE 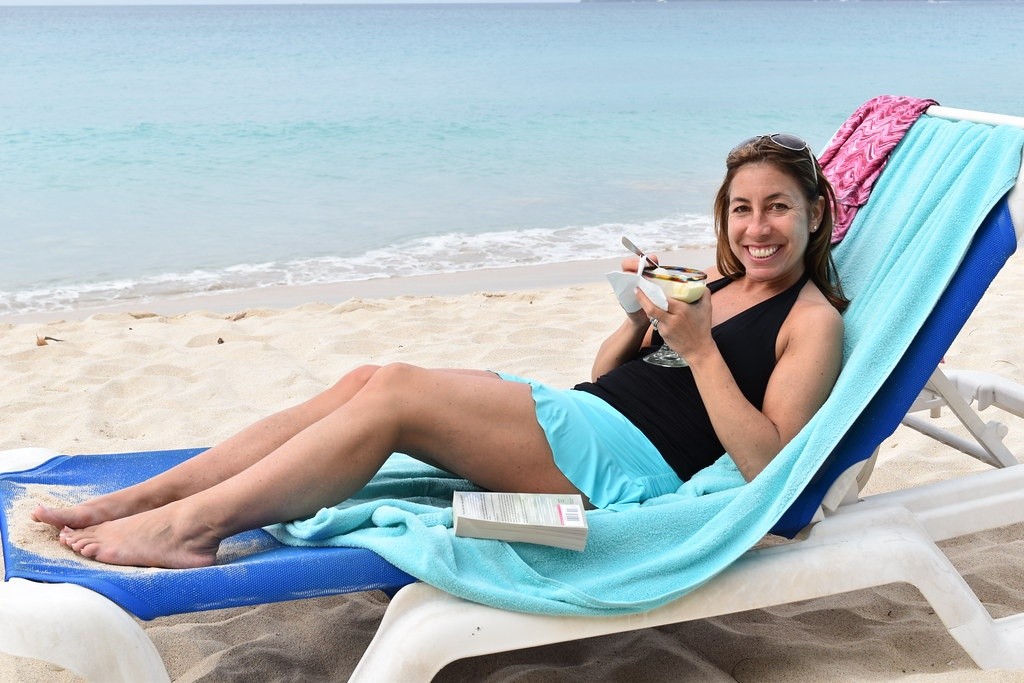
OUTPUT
[648,316,660,331]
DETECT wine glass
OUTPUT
[640,266,708,369]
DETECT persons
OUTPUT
[23,130,855,571]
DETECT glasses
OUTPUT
[729,133,818,186]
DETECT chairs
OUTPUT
[0,88,1024,682]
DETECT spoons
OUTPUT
[621,236,661,269]
